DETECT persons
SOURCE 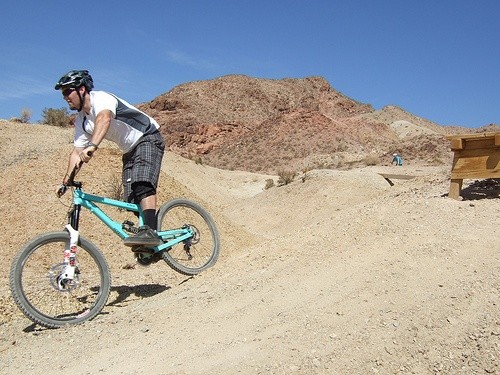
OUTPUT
[55,70,165,259]
[391,153,402,166]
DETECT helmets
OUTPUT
[393,153,397,156]
[54,70,94,93]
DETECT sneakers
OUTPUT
[124,225,161,246]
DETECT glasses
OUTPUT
[61,88,76,96]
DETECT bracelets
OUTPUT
[84,141,98,150]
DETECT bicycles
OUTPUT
[9,151,221,330]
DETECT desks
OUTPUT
[447,131,500,199]
